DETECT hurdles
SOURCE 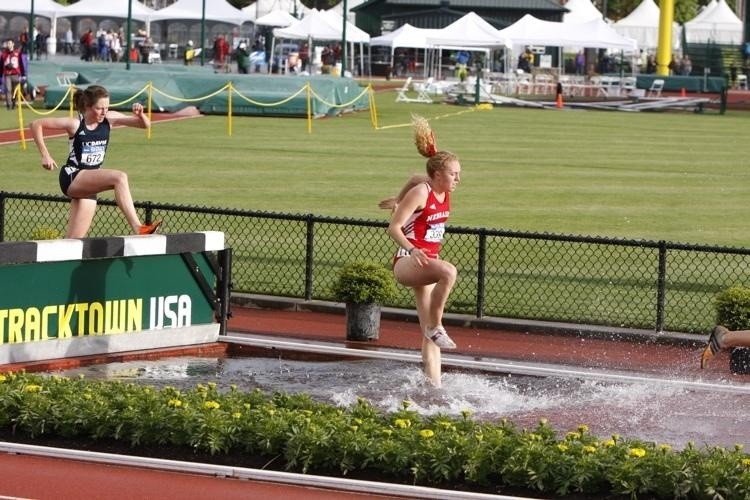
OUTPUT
[0,230,233,365]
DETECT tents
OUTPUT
[269,8,372,78]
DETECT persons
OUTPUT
[183,31,346,76]
[376,112,463,391]
[700,321,750,370]
[1,39,30,110]
[600,51,611,74]
[78,25,153,63]
[63,28,74,54]
[648,56,656,73]
[745,54,750,91]
[639,49,649,74]
[19,26,48,59]
[668,54,681,75]
[28,83,163,242]
[575,49,585,76]
[456,49,470,82]
[520,45,535,72]
[681,54,693,76]
[728,58,740,89]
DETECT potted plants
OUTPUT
[328,259,398,343]
[713,286,750,375]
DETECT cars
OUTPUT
[249,43,300,71]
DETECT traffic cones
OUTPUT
[556,93,564,108]
[680,87,687,97]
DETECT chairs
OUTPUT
[393,67,665,104]
[50,29,180,65]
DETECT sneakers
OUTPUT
[701,326,728,370]
[424,324,456,349]
[140,220,162,234]
